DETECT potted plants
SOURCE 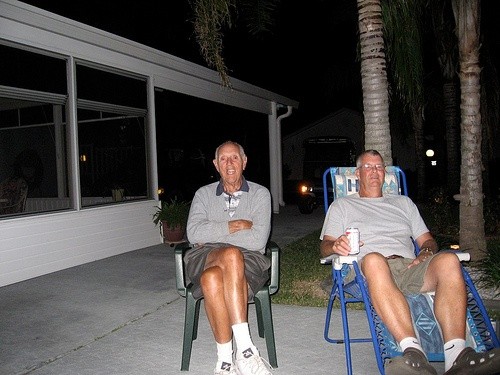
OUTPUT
[150,196,192,242]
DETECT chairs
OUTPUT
[174,241,279,370]
[0,177,28,215]
[320,166,500,375]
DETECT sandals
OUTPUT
[443,347,500,375]
[384,347,438,375]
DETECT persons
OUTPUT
[184,141,275,374]
[319,150,500,375]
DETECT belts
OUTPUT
[386,255,405,260]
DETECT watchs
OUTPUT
[417,247,434,258]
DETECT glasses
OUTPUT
[356,163,384,171]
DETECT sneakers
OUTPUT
[214,347,274,375]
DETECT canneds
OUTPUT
[345,227,360,255]
